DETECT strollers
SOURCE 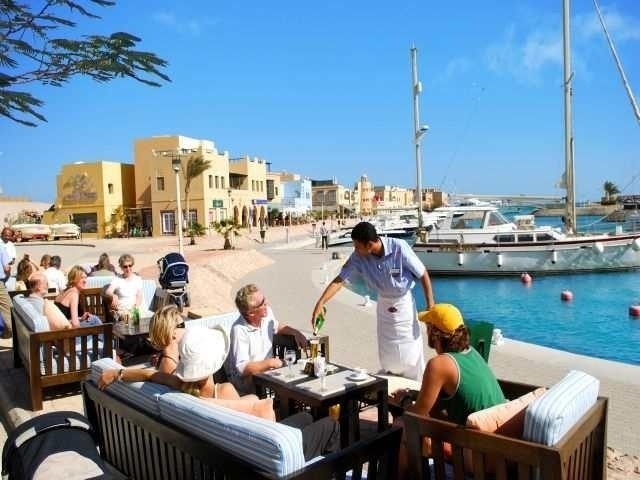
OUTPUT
[156,253,191,311]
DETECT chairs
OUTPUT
[403,379,609,480]
[78,283,202,324]
[167,311,332,378]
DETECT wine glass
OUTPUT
[313,357,329,394]
[283,350,297,375]
[113,309,122,327]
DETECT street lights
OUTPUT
[172,157,185,259]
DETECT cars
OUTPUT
[9,220,81,242]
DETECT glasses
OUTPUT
[175,320,186,328]
[253,296,265,309]
[121,264,133,268]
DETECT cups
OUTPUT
[304,336,319,359]
[354,369,368,377]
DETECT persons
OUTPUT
[96,323,341,463]
[105,253,144,322]
[222,282,308,396]
[311,221,433,383]
[17,252,117,300]
[389,304,507,480]
[53,265,115,349]
[0,237,14,337]
[0,227,15,336]
[146,304,185,374]
[319,222,328,250]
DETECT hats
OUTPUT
[418,304,465,338]
[175,322,234,383]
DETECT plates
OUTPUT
[348,374,369,380]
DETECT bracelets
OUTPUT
[117,369,124,382]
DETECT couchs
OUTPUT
[8,288,113,412]
[80,380,403,480]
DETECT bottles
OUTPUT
[312,305,326,336]
[132,304,140,327]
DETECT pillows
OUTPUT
[518,369,600,480]
[86,276,157,305]
[185,306,286,356]
[90,357,171,418]
[12,293,54,362]
[159,392,307,478]
[463,387,546,472]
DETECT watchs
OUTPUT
[399,394,411,404]
